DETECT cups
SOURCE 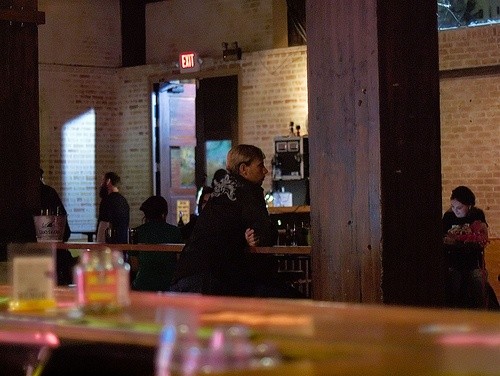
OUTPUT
[74,248,130,313]
[33,203,66,243]
[7,242,57,315]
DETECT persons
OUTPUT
[174,144,306,298]
[210,169,228,187]
[97,172,129,262]
[177,187,214,269]
[22,169,76,286]
[442,186,488,309]
[133,196,182,292]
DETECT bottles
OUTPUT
[285,221,311,246]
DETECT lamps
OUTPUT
[160,82,178,92]
[221,40,242,60]
